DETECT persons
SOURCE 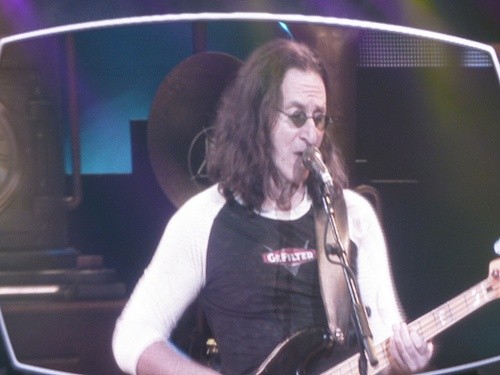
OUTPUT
[111,39,435,375]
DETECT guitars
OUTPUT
[240,268,500,375]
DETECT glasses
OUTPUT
[277,107,333,131]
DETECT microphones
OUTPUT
[302,146,335,192]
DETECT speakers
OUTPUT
[1,58,71,253]
